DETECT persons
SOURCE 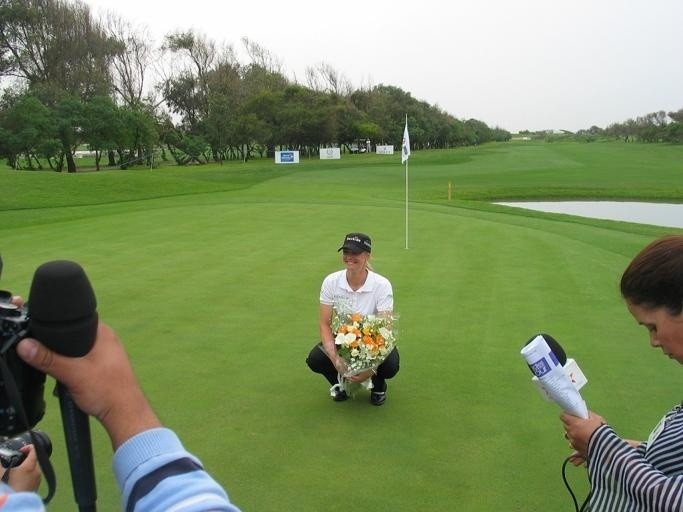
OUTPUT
[306,231,400,405]
[560,234,683,512]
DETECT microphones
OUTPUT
[524,334,588,401]
[24,260,98,512]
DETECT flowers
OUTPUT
[331,305,392,400]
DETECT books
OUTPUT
[519,333,589,419]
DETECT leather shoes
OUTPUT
[332,385,352,402]
[369,390,387,407]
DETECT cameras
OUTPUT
[0,428,52,472]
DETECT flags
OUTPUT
[401,122,411,164]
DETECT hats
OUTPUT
[337,232,372,255]
